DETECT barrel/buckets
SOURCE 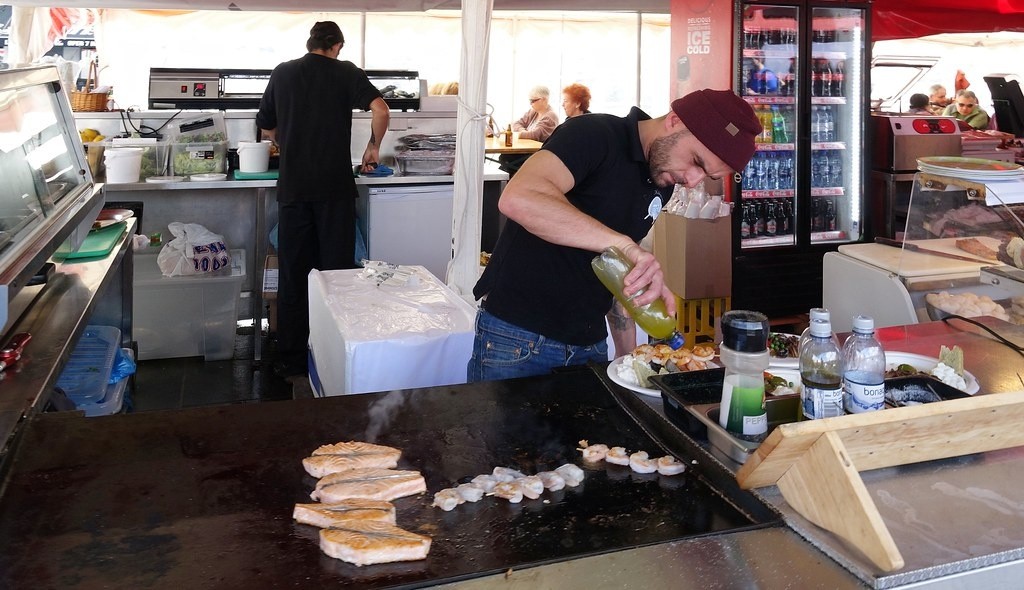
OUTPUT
[236,140,272,173]
[103,147,144,183]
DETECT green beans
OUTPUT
[770,337,785,351]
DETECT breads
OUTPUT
[938,344,964,378]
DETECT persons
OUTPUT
[929,84,957,116]
[255,21,390,363]
[942,90,990,129]
[975,98,999,131]
[499,86,559,172]
[562,84,592,121]
[466,88,763,384]
[908,93,938,116]
[428,82,458,95]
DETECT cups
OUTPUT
[662,184,733,220]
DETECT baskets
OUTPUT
[70,62,108,111]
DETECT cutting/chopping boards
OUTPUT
[838,236,1006,277]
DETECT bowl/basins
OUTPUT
[647,368,775,441]
[923,291,960,322]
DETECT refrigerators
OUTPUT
[668,0,872,332]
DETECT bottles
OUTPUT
[718,310,770,442]
[487,119,493,137]
[840,315,885,414]
[505,124,513,147]
[741,5,798,240]
[810,7,847,232]
[799,307,840,354]
[591,247,686,352]
[799,318,844,422]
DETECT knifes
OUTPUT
[874,236,998,266]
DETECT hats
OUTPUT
[911,94,929,107]
[671,89,763,173]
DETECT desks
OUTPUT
[485,132,543,171]
[0,217,138,453]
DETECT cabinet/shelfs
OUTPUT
[865,168,919,245]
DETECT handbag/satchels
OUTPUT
[156,223,231,277]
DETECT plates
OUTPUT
[916,156,1024,181]
[768,332,801,368]
[884,376,973,408]
[606,354,720,399]
[884,350,980,395]
[762,368,801,396]
[1006,145,1024,152]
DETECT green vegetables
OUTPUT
[170,131,227,174]
[139,146,158,179]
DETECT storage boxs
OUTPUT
[81,136,110,177]
[55,325,134,416]
[171,139,229,175]
[136,248,246,360]
[654,211,731,298]
[420,79,457,112]
[308,265,478,398]
[394,155,455,175]
[101,135,170,177]
[167,111,227,143]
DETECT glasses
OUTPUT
[956,101,975,108]
[530,99,539,104]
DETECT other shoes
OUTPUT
[269,359,305,379]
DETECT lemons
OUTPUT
[79,128,105,175]
